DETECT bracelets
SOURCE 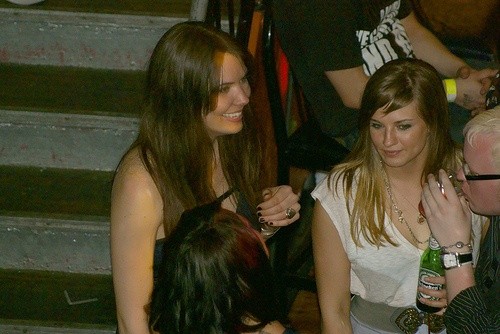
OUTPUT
[444,78,457,102]
[439,239,472,253]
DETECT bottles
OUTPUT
[416,234,445,313]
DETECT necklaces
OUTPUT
[392,183,425,223]
[384,175,431,244]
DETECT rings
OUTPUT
[287,207,296,219]
[445,301,448,307]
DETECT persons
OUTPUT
[419,102,500,334]
[109,21,300,334]
[148,204,302,334]
[272,0,500,155]
[307,58,490,334]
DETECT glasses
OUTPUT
[460,154,500,183]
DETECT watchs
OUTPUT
[441,252,473,270]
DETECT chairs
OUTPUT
[261,11,352,324]
